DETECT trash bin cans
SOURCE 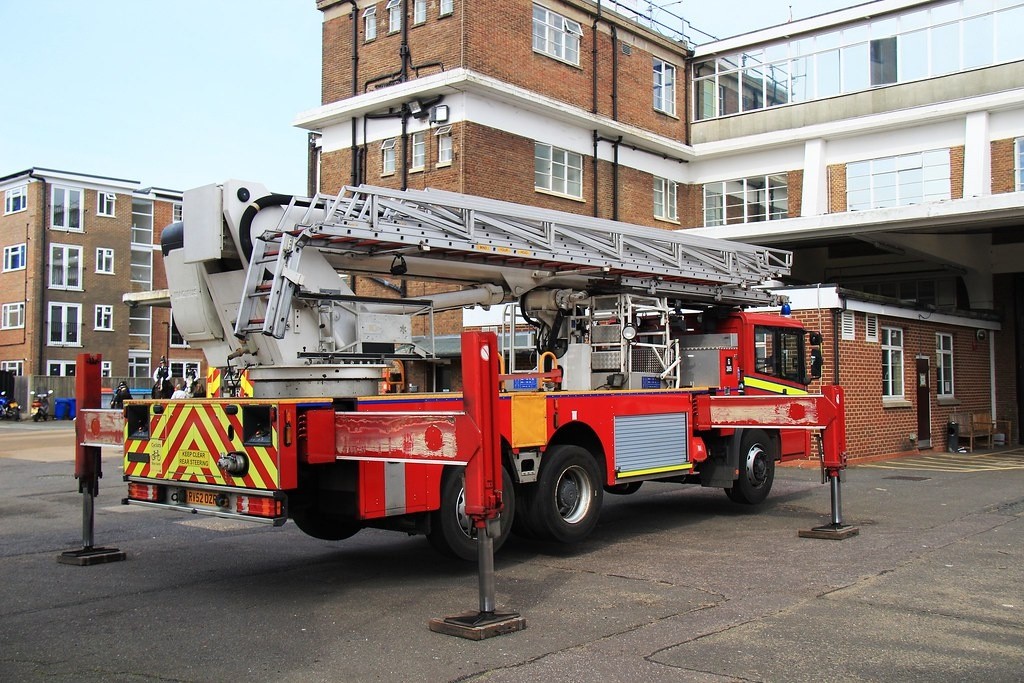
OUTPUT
[55,398,68,420]
[68,398,76,419]
[946,423,959,454]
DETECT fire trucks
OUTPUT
[57,182,862,641]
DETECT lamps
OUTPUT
[607,373,624,389]
[408,99,429,119]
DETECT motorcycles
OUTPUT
[0,391,21,421]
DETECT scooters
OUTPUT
[29,390,53,421]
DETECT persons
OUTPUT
[110,381,133,409]
[153,359,205,399]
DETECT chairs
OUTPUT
[948,411,1010,453]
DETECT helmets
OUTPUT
[160,355,166,363]
[186,365,191,368]
[117,382,126,388]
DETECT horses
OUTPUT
[151,371,205,403]
